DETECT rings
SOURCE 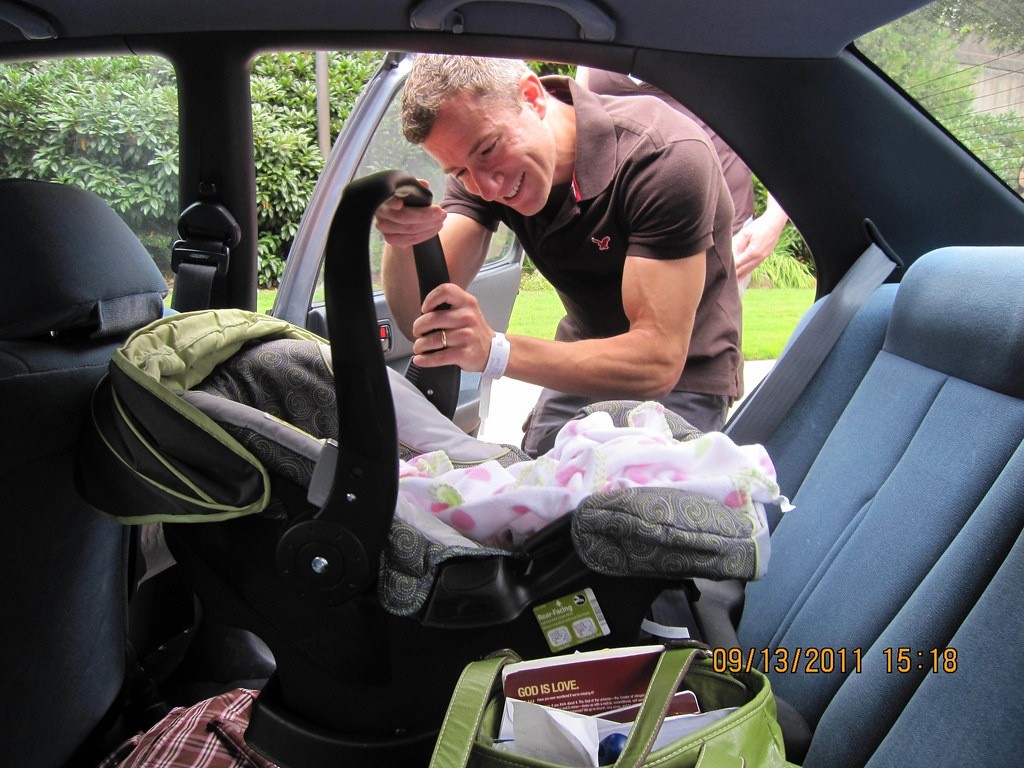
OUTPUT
[440,329,448,348]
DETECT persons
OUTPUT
[371,53,790,465]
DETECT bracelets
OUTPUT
[482,331,511,381]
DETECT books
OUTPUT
[498,643,670,719]
